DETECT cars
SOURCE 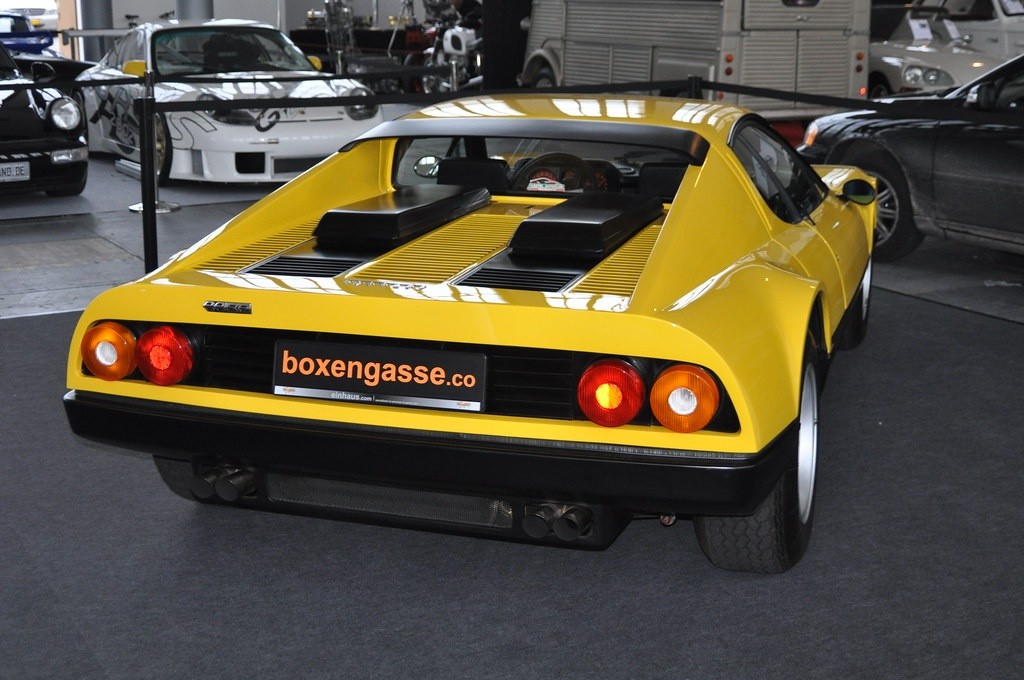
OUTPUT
[797,54,1024,261]
[0,12,100,202]
[910,1,1024,60]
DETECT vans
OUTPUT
[515,1,872,100]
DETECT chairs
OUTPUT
[436,157,512,191]
[635,160,691,201]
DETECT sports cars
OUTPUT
[70,17,385,185]
[61,93,878,574]
[868,6,1006,99]
